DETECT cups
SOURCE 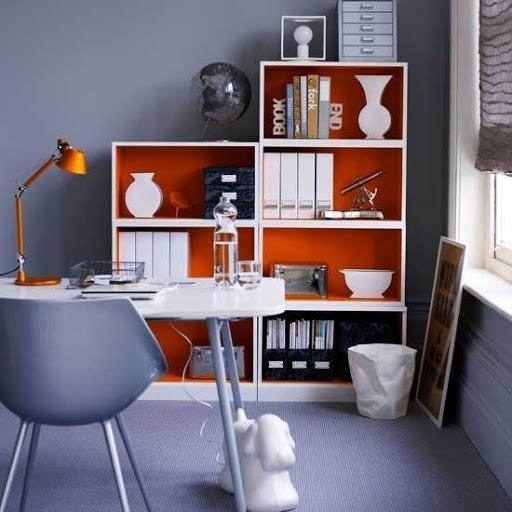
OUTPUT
[238,260,262,290]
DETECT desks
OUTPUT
[1,273,289,512]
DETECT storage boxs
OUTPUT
[189,344,246,379]
[338,1,398,64]
[203,166,255,220]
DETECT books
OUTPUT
[262,74,334,221]
[265,318,335,351]
[81,231,189,301]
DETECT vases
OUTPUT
[123,170,164,219]
[352,73,395,139]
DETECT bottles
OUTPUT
[125,173,162,218]
[213,197,238,289]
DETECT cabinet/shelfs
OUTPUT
[110,140,258,405]
[259,60,410,405]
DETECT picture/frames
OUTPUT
[415,235,470,430]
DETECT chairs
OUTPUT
[1,293,171,512]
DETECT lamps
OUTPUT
[14,138,90,288]
[279,15,327,61]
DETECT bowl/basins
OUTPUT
[339,269,395,299]
